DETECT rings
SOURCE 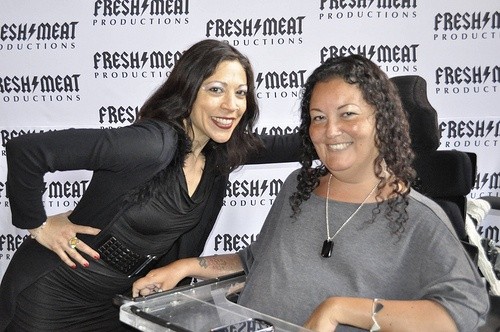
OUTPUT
[69,237,79,248]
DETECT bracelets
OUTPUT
[30,220,47,239]
[370,298,383,332]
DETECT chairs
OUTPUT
[390,75,479,263]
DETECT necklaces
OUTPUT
[322,175,380,256]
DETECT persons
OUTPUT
[133,55,490,332]
[0,40,319,332]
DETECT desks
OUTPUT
[118,271,312,332]
